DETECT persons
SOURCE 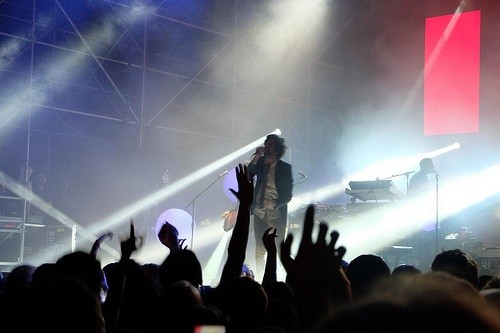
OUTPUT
[154,163,255,316]
[247,134,293,279]
[407,157,439,268]
[0,205,500,333]
[223,200,240,232]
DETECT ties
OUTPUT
[258,165,271,208]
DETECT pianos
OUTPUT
[344,180,405,201]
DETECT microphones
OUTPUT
[253,147,269,155]
[220,171,228,177]
[296,169,308,180]
